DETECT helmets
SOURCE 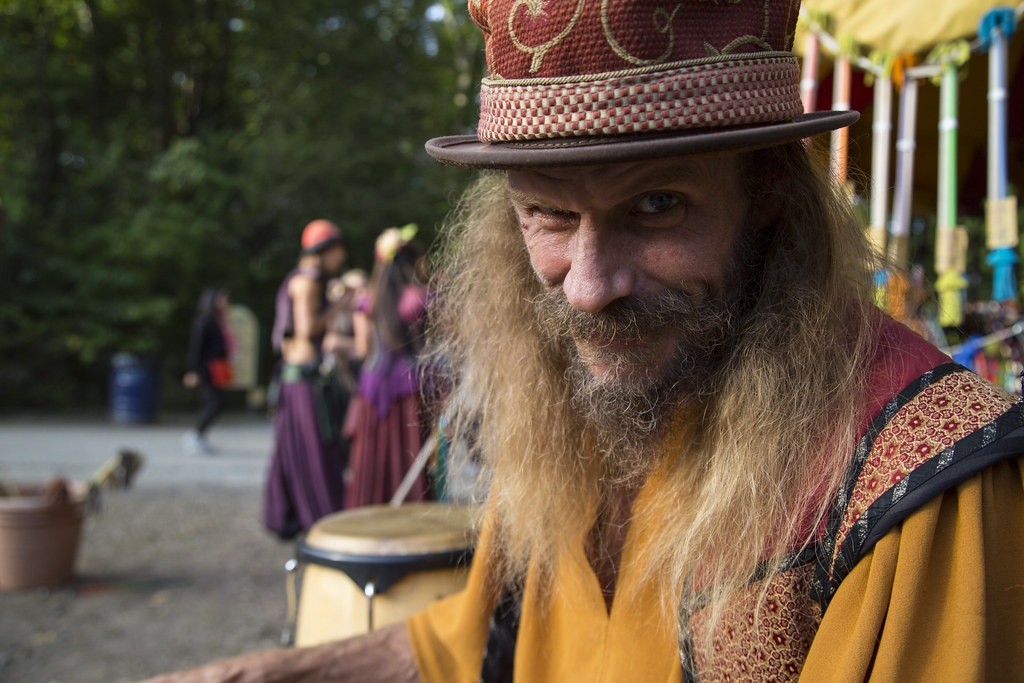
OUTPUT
[372,225,414,264]
[300,217,342,255]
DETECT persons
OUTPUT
[339,222,430,511]
[264,222,365,538]
[187,288,227,450]
[133,0,1023,683]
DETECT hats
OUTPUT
[426,1,864,169]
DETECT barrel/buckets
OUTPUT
[0,484,84,591]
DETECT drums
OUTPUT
[288,503,482,650]
